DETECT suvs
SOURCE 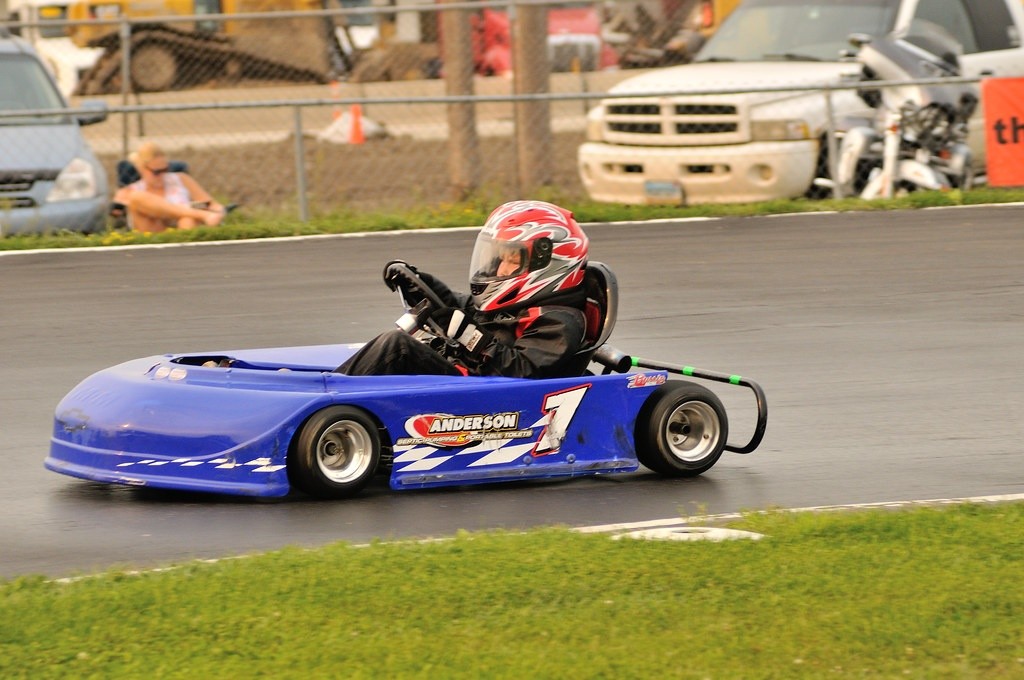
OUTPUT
[575,1,1024,207]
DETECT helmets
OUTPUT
[468,200,589,323]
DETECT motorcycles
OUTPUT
[817,28,993,201]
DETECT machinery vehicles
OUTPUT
[63,1,435,124]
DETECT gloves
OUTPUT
[382,258,431,299]
[432,306,486,355]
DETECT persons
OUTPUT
[338,201,590,378]
[115,142,226,232]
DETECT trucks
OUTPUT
[433,0,621,82]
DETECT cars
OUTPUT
[0,25,113,237]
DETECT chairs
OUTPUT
[109,159,238,234]
[536,260,621,380]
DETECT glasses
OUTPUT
[146,166,170,176]
